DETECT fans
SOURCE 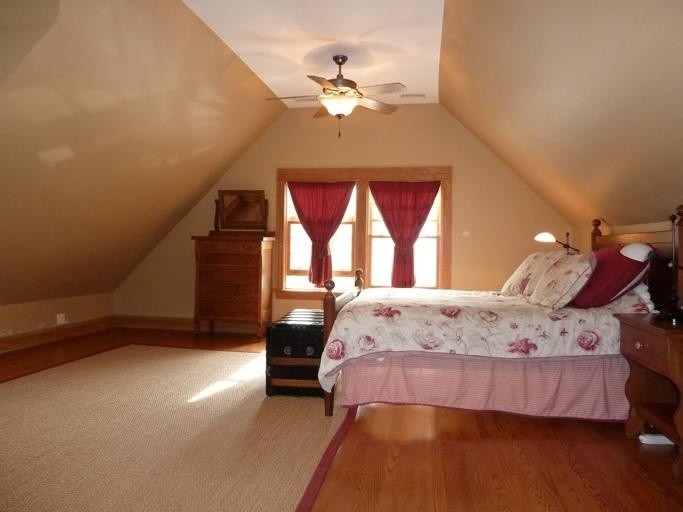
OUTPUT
[264,54,406,119]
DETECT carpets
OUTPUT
[0,344,358,512]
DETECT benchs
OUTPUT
[264,308,324,397]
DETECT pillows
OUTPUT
[497,243,653,310]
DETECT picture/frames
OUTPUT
[217,189,265,229]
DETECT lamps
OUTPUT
[533,231,579,253]
[619,215,682,323]
[318,96,359,138]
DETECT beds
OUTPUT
[323,204,682,420]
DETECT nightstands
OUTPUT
[612,312,682,483]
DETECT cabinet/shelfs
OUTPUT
[191,231,275,341]
